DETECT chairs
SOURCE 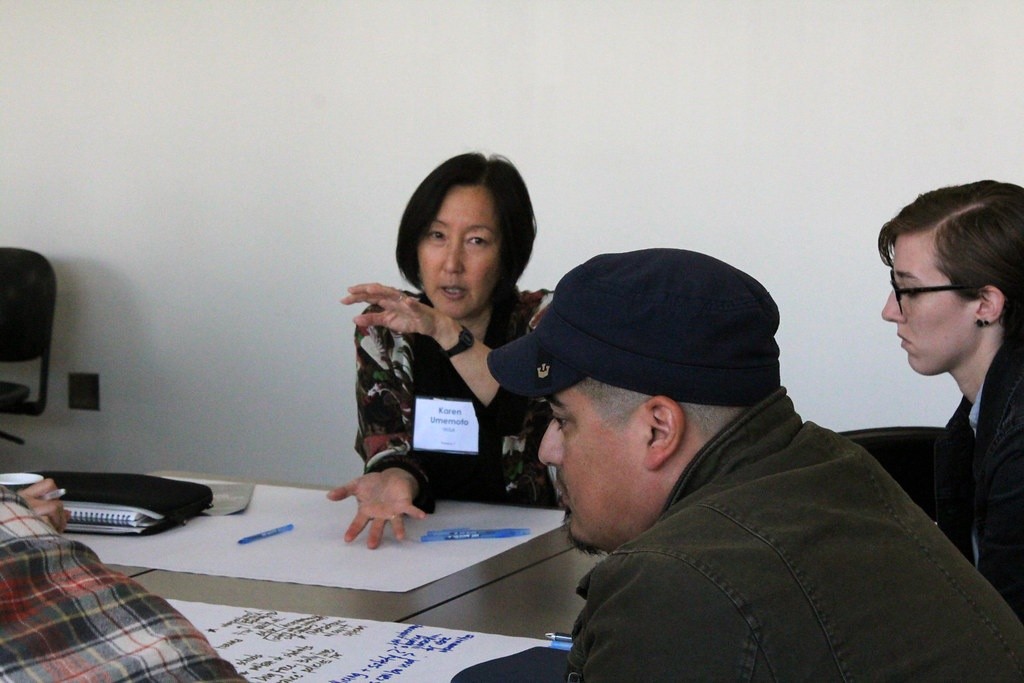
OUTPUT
[0,247,57,445]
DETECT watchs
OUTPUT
[444,324,474,359]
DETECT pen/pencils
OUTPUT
[40,488,66,500]
[545,632,572,643]
[237,524,293,545]
[421,528,531,542]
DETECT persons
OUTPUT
[878,179,1024,628]
[326,152,563,550]
[487,249,1024,683]
[0,477,251,683]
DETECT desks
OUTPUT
[60,469,606,641]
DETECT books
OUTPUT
[61,506,155,532]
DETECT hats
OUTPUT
[484,246,780,408]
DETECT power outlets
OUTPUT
[67,372,101,413]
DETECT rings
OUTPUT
[389,514,402,522]
[396,293,404,303]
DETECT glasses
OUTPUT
[889,269,981,315]
